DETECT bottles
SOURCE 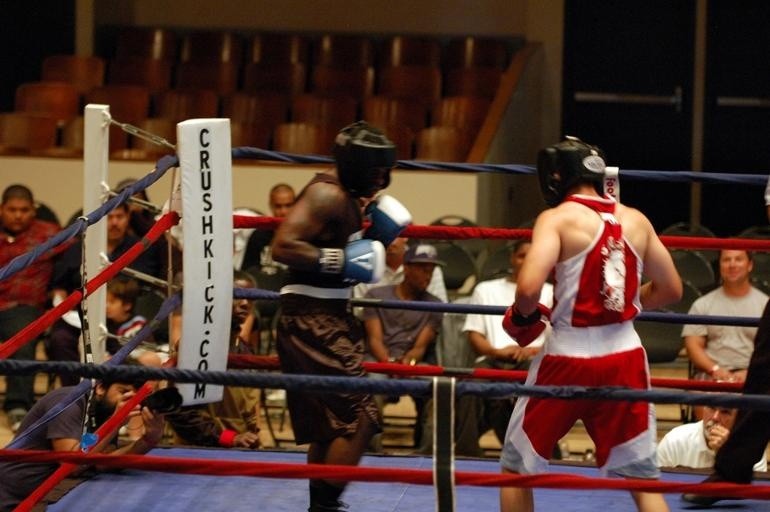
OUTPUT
[559,439,571,460]
[582,448,593,461]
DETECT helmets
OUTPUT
[535,137,603,209]
[332,120,397,197]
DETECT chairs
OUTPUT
[425,214,534,369]
[631,224,725,367]
[735,225,767,287]
[2,32,503,171]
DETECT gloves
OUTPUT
[361,193,412,248]
[317,238,387,285]
[500,302,546,348]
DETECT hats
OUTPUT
[401,244,448,268]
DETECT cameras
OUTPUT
[140,385,184,414]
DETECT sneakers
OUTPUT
[8,405,26,433]
